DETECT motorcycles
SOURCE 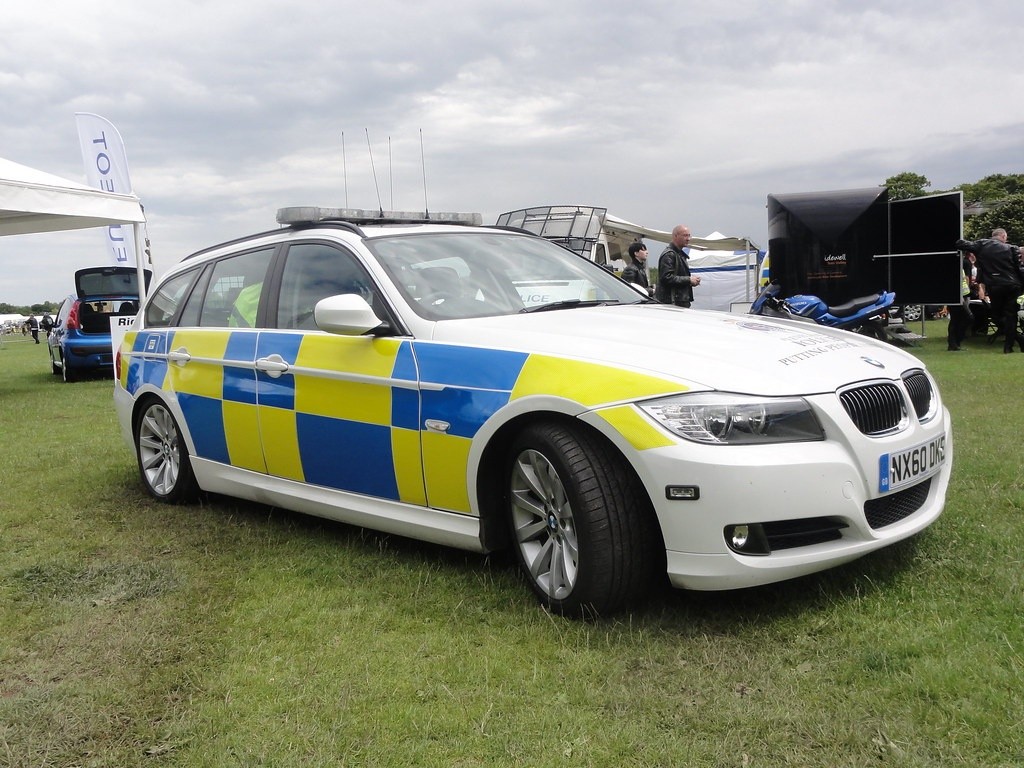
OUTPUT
[746,276,897,344]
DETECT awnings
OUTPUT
[608,219,760,302]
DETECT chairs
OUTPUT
[119,302,134,313]
[967,299,997,333]
[415,265,459,305]
[84,304,94,314]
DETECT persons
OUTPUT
[655,224,701,308]
[42,312,54,333]
[24,314,40,344]
[620,242,656,297]
[941,228,1024,353]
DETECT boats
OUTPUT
[493,204,767,315]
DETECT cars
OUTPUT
[116,205,955,620]
[41,266,153,383]
[889,305,944,322]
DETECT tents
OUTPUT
[0,157,147,303]
